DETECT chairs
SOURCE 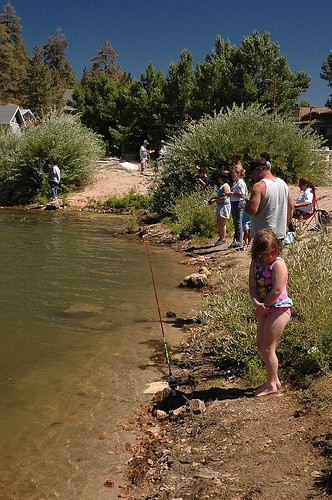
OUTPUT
[292,194,323,232]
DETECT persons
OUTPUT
[244,152,293,249]
[293,177,314,216]
[195,152,273,244]
[138,139,155,174]
[249,228,293,398]
[224,168,245,248]
[208,172,232,246]
[50,160,61,201]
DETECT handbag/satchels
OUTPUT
[318,210,332,225]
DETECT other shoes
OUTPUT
[229,241,244,249]
[214,238,226,245]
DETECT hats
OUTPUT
[143,139,151,144]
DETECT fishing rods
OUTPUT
[145,237,177,390]
[130,201,208,237]
